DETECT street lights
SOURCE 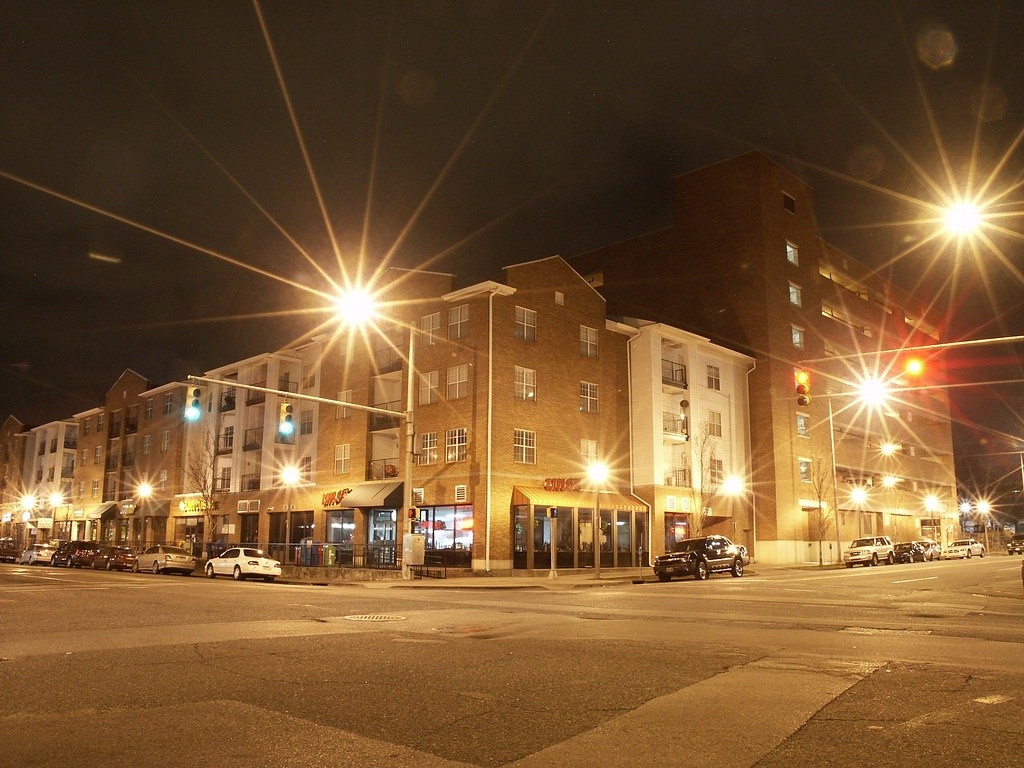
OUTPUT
[138,483,151,553]
[590,461,608,579]
[925,496,991,555]
[828,381,881,563]
[341,291,416,579]
[282,464,299,564]
[21,493,61,548]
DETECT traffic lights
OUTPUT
[547,507,558,518]
[795,368,811,406]
[185,386,201,418]
[279,402,292,432]
[906,356,923,376]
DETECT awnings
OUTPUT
[341,482,403,508]
[90,503,116,520]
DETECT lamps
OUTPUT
[676,413,685,421]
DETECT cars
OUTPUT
[90,545,281,582]
[894,539,985,563]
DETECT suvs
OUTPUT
[50,541,98,568]
[0,538,17,563]
[19,544,55,565]
[1007,535,1024,555]
[844,535,895,568]
[654,535,750,580]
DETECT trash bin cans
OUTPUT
[318,543,327,566]
[324,546,336,566]
[300,537,313,566]
[294,546,299,566]
[312,543,318,566]
